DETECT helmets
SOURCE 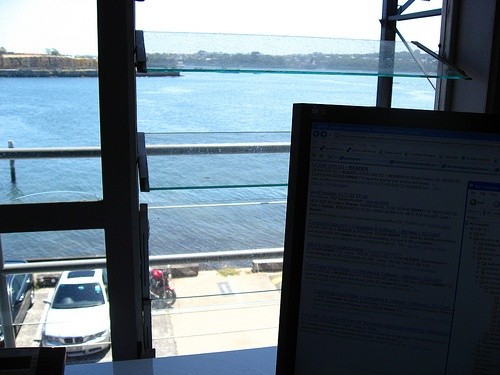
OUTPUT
[151,268,162,281]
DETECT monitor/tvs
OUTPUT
[276,104,500,375]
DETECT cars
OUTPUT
[38,270,111,364]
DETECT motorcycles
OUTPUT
[145,268,176,307]
